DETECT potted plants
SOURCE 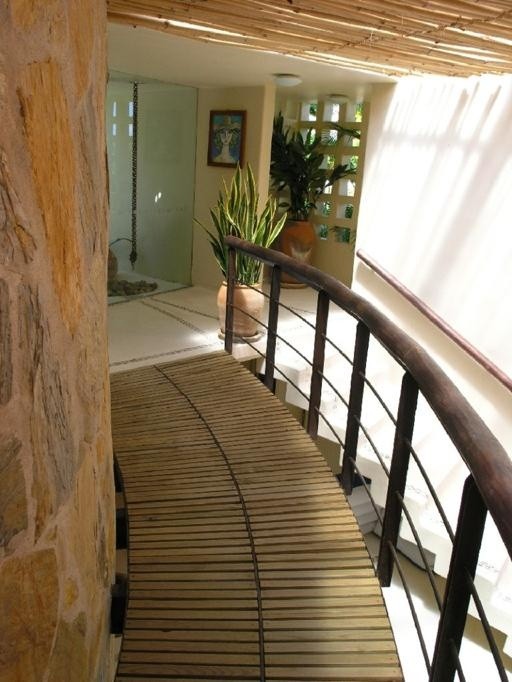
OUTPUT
[267,108,358,289]
[191,161,288,343]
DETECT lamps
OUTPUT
[272,73,302,87]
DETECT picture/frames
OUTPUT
[207,108,247,169]
